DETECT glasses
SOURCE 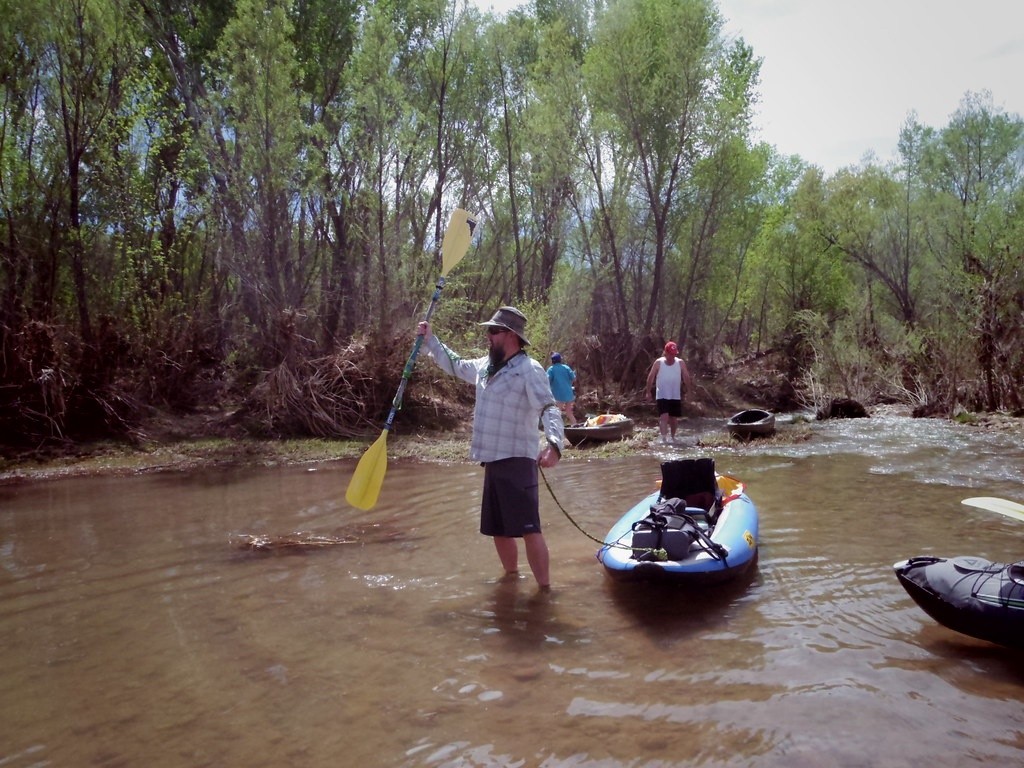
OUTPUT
[488,327,510,334]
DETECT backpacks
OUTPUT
[632,503,730,563]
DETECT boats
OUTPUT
[892,553,1024,651]
[728,408,776,437]
[599,474,762,589]
[563,418,635,446]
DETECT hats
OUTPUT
[479,306,532,346]
[551,354,560,359]
[666,341,678,355]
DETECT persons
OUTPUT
[543,349,582,429]
[415,307,565,606]
[644,340,693,448]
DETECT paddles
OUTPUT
[345,208,479,512]
